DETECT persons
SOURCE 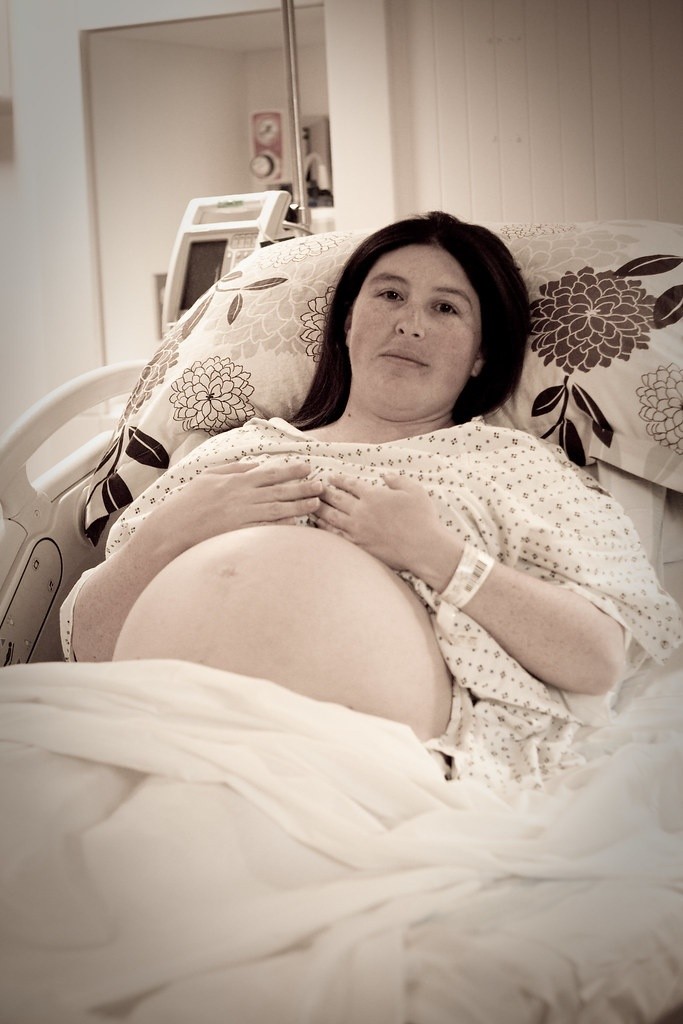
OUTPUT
[0,220,683,1024]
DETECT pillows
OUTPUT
[83,219,683,548]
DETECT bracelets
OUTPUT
[437,544,497,611]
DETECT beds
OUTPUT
[0,219,683,1024]
